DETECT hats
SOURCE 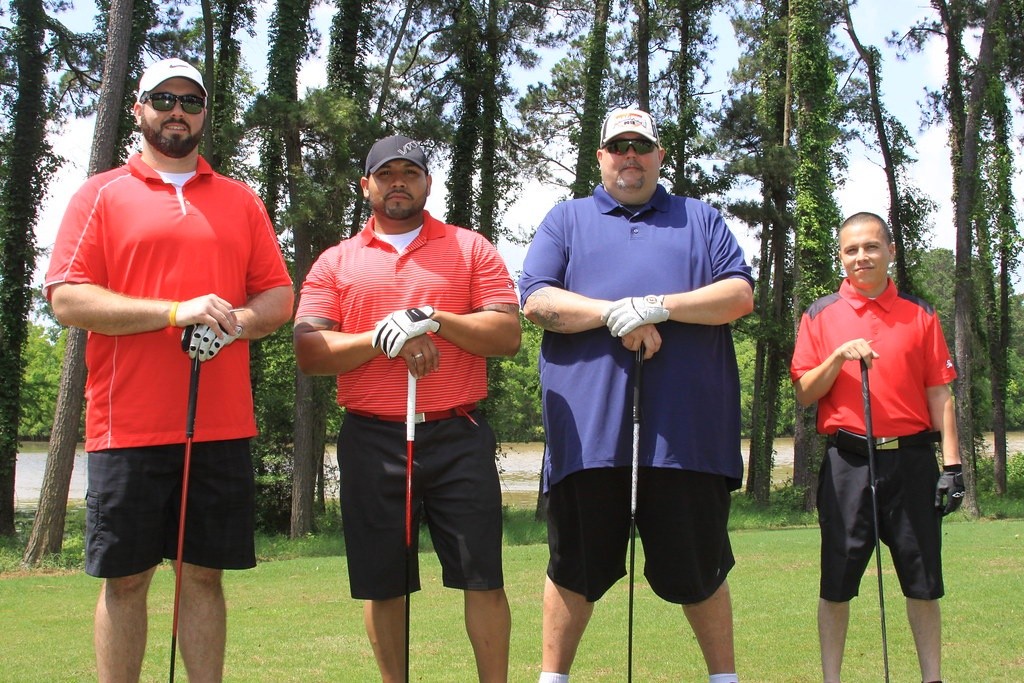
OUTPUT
[365,136,429,175]
[138,58,208,107]
[599,108,660,147]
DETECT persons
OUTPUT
[518,110,758,683]
[790,212,965,683]
[42,58,293,683]
[294,134,521,681]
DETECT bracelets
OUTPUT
[170,301,179,326]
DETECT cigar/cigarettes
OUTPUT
[230,309,244,312]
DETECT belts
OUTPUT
[346,403,480,426]
[827,427,942,456]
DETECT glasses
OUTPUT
[142,92,205,114]
[604,139,655,155]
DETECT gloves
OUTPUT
[601,295,670,337]
[371,304,441,359]
[934,464,966,516]
[180,322,244,362]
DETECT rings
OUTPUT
[415,353,422,358]
